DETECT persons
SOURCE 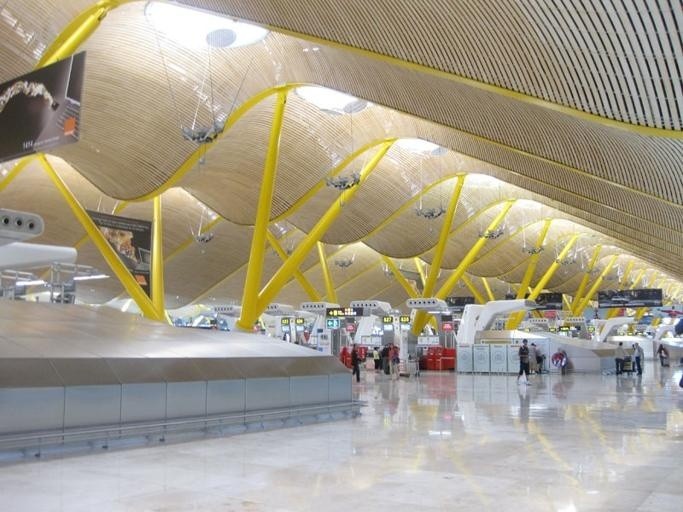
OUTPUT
[350,343,400,383]
[515,339,568,385]
[614,341,644,376]
[655,344,670,367]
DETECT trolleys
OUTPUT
[622,355,636,375]
[396,355,421,378]
